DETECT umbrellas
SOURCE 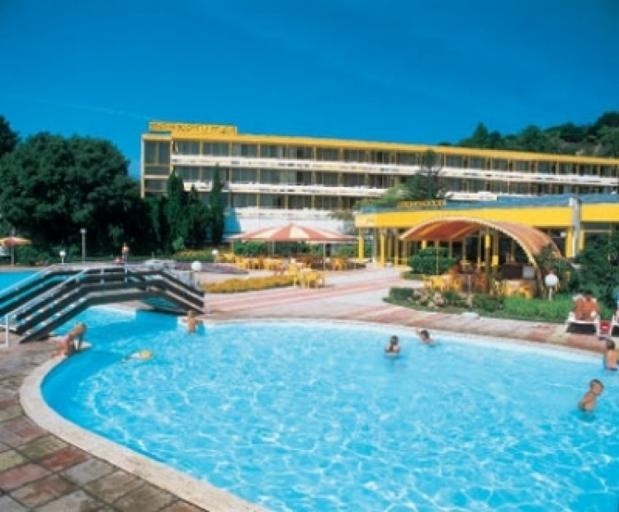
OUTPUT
[224,224,357,269]
[0,236,32,265]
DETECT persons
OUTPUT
[384,336,400,353]
[605,340,619,369]
[181,309,203,334]
[580,380,603,413]
[121,242,130,265]
[545,268,559,301]
[417,328,432,344]
[575,291,599,321]
[51,323,86,358]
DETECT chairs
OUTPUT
[560,296,602,336]
